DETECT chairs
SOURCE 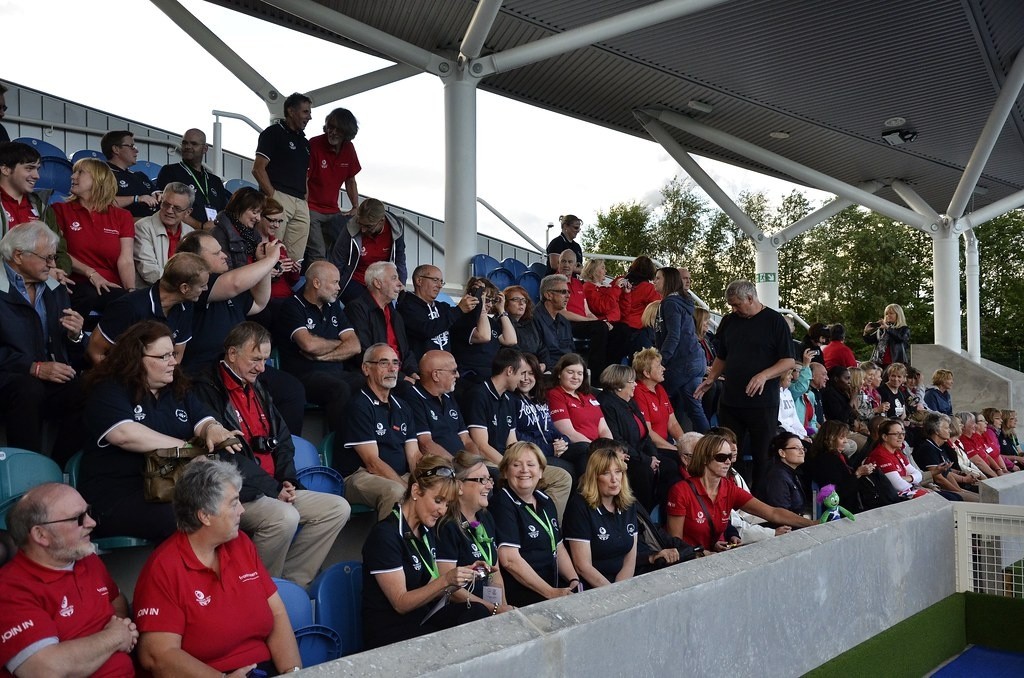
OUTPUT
[0,138,755,678]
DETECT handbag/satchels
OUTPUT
[850,465,878,498]
[142,436,241,502]
[908,397,930,422]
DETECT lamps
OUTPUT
[881,130,918,146]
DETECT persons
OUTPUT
[666,434,819,560]
[562,447,638,596]
[0,483,140,678]
[0,94,1024,678]
[435,449,521,612]
[487,441,581,608]
[132,460,304,678]
[361,453,498,651]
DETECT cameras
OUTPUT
[472,566,487,580]
[251,436,279,455]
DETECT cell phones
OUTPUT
[474,285,484,300]
[808,350,820,355]
[296,259,304,265]
[870,323,881,327]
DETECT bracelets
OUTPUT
[491,602,499,616]
[569,579,579,583]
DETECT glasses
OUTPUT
[366,360,402,369]
[180,142,206,148]
[547,289,570,295]
[627,380,635,385]
[509,297,528,304]
[438,369,458,375]
[794,368,801,373]
[462,477,495,485]
[143,352,178,361]
[421,275,446,286]
[0,104,8,112]
[416,466,456,479]
[355,219,381,230]
[572,226,582,232]
[161,202,191,214]
[887,431,906,438]
[40,506,92,527]
[25,249,58,263]
[121,143,137,150]
[712,453,733,462]
[976,419,986,423]
[784,446,807,453]
[485,295,498,304]
[265,217,284,224]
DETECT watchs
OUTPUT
[283,666,299,674]
